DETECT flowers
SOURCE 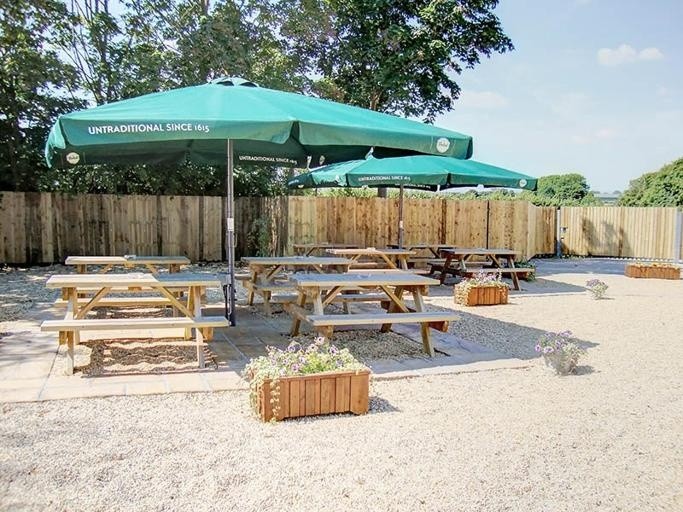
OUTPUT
[243,337,359,423]
[534,329,586,355]
[586,278,608,291]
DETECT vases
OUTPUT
[624,264,680,279]
[589,291,602,299]
[544,355,577,374]
[250,363,372,421]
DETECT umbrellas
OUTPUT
[284,154,539,267]
[40,75,475,328]
